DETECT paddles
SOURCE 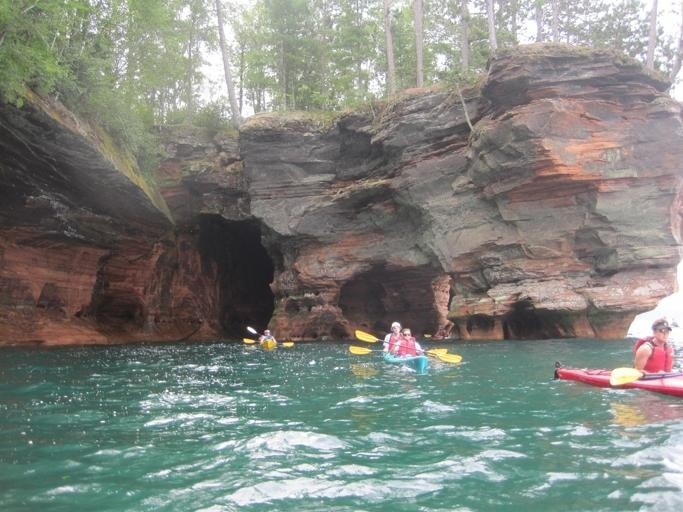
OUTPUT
[349,345,448,356]
[355,329,463,364]
[243,338,294,347]
[247,326,264,337]
[610,366,682,389]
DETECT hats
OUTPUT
[654,322,672,331]
[391,322,402,331]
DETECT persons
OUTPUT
[388,327,425,358]
[631,318,674,376]
[259,329,276,345]
[380,321,403,353]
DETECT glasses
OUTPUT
[403,334,411,336]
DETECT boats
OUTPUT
[261,339,276,349]
[383,352,430,375]
[549,360,683,398]
